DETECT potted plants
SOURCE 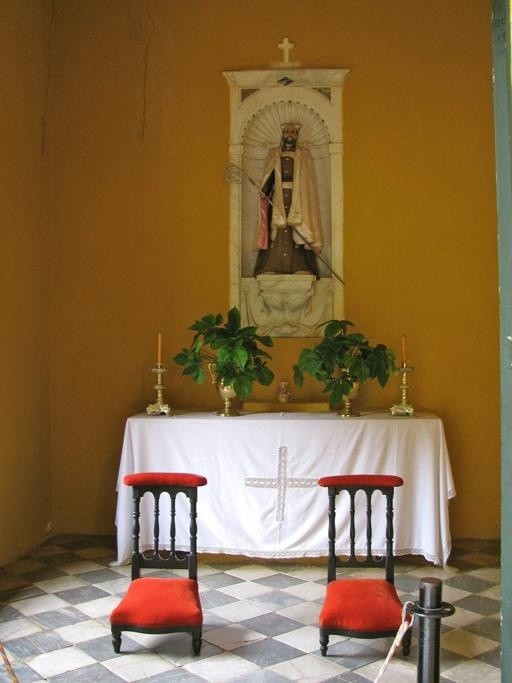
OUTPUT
[173,305,274,416]
[294,317,398,415]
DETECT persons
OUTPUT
[254,122,324,281]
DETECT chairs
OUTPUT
[109,472,209,654]
[318,474,413,655]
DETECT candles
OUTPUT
[157,332,162,362]
[401,334,406,361]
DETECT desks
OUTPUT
[116,411,455,570]
